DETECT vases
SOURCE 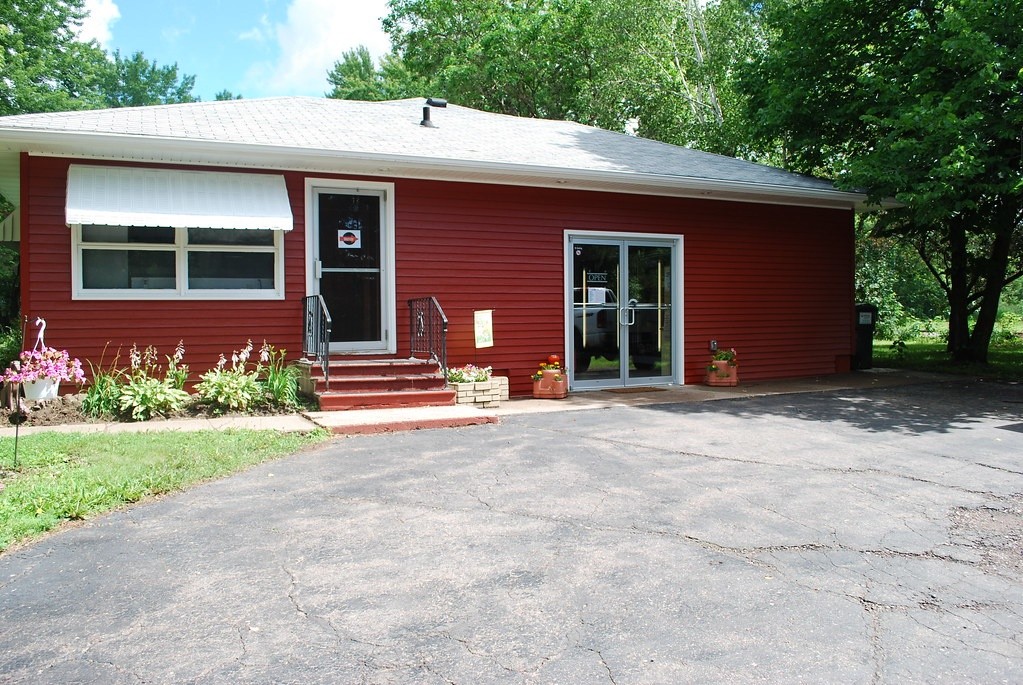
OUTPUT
[22,375,60,400]
[708,360,737,387]
[450,381,500,408]
[534,370,568,399]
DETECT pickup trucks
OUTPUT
[574,287,663,373]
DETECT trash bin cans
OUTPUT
[854,301,878,372]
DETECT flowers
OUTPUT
[0,346,86,386]
[444,364,493,382]
[707,346,738,377]
[530,353,569,382]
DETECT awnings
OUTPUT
[64,163,294,234]
